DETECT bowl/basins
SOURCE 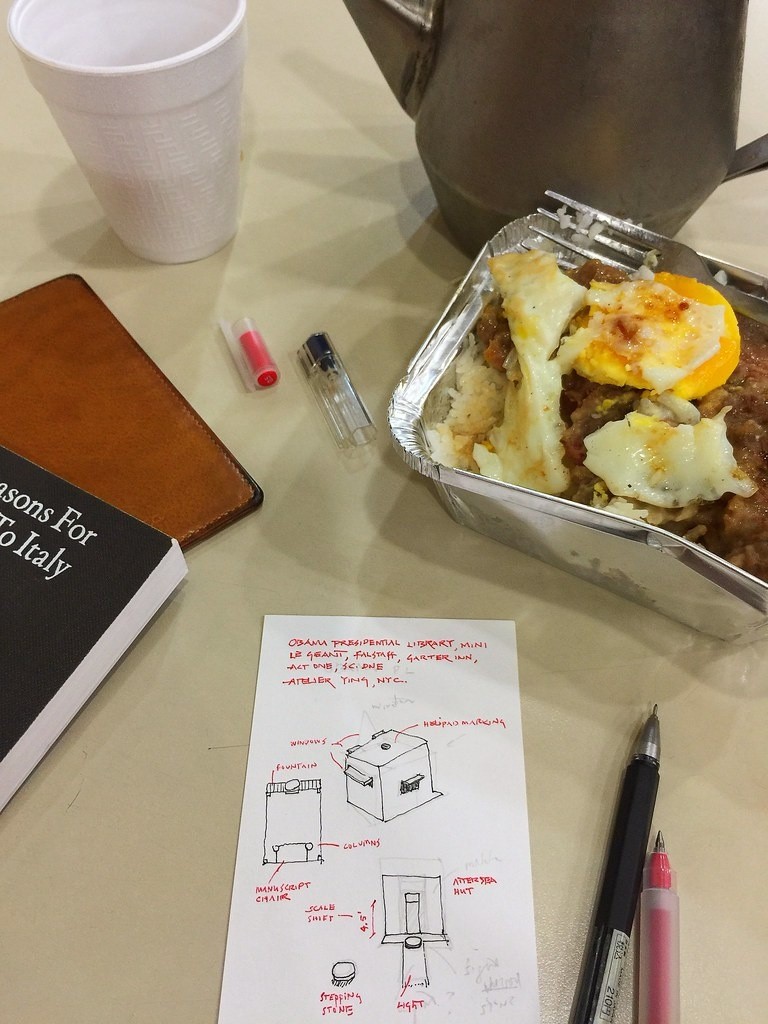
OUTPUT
[387,211,768,642]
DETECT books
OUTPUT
[0,447,190,812]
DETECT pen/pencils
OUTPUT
[638,827,683,1024]
[562,700,662,1024]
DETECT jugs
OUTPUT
[343,0,767,257]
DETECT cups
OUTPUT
[6,0,248,267]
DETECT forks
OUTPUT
[518,190,768,338]
